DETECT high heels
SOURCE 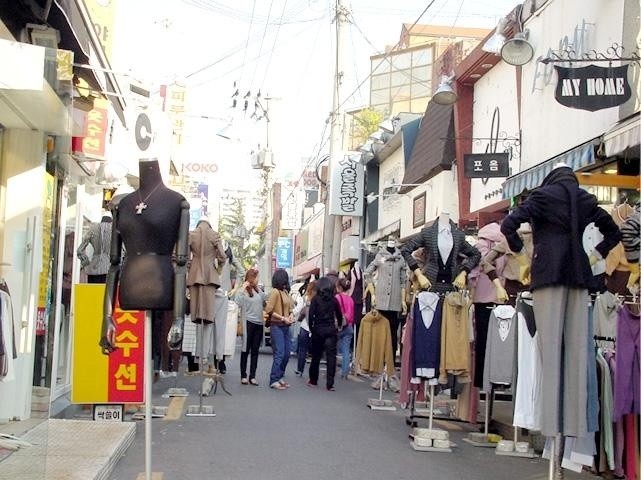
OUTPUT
[294,369,302,376]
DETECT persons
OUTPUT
[183,217,226,325]
[400,212,481,298]
[97,155,190,354]
[499,162,622,437]
[363,239,408,364]
[213,253,363,395]
[466,220,507,389]
[75,211,114,283]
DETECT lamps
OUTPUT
[498,27,536,67]
[73,62,149,98]
[383,179,432,194]
[432,67,460,106]
[366,191,412,203]
[72,86,147,113]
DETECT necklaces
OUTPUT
[135,180,164,215]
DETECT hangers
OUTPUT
[425,282,433,296]
[452,282,461,298]
[371,305,378,316]
[591,334,618,351]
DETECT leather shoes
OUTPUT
[241,377,248,384]
[249,377,259,386]
[270,382,286,390]
[279,379,290,388]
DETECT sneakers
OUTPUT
[325,383,336,390]
[306,379,317,387]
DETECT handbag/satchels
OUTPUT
[335,316,347,330]
[265,312,272,329]
[298,307,305,321]
